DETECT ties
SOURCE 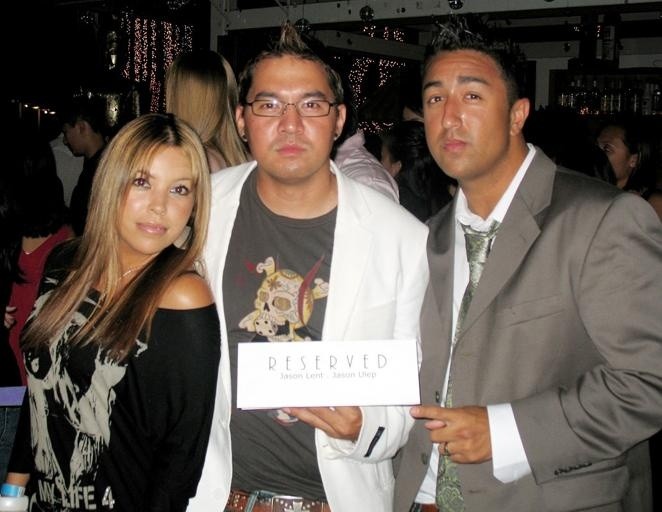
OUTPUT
[435,232,502,512]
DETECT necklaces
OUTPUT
[96,265,140,301]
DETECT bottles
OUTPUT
[558,79,662,116]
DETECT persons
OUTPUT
[528,104,660,216]
[171,20,431,511]
[331,106,458,224]
[0,90,118,491]
[0,111,222,511]
[163,46,253,174]
[389,13,661,512]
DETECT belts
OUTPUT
[410,502,439,512]
[225,491,331,512]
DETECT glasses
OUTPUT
[244,100,340,118]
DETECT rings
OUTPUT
[443,442,451,457]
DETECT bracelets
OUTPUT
[0,482,26,498]
